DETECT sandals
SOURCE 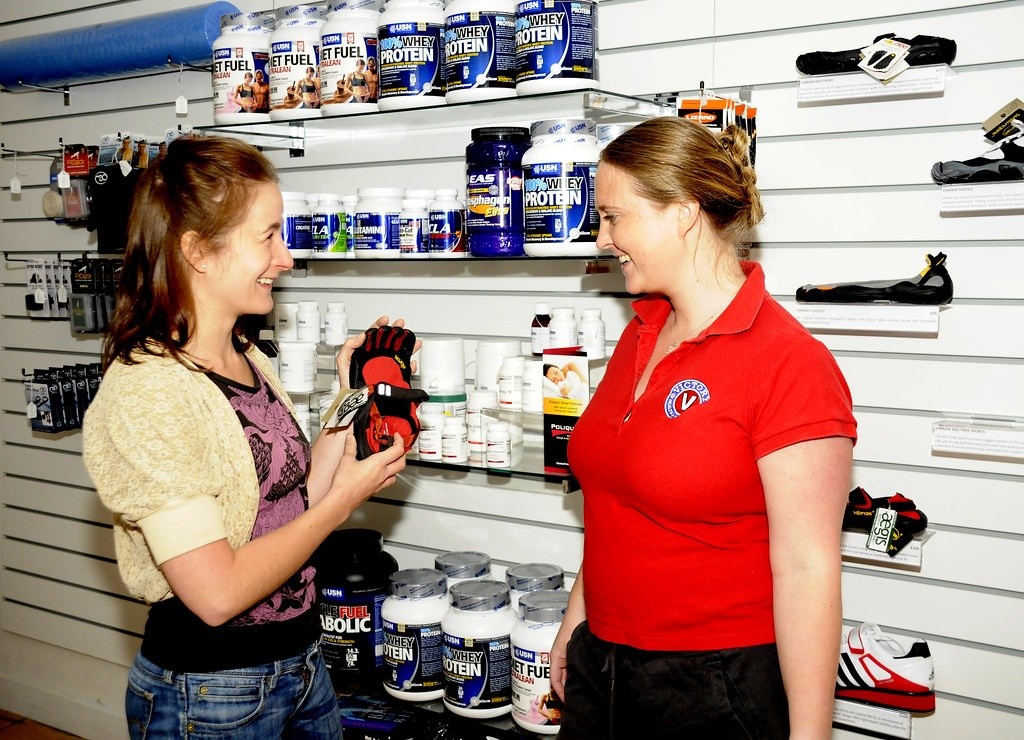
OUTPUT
[842,487,928,538]
[348,325,429,462]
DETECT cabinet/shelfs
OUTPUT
[189,88,679,740]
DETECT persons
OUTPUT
[81,136,406,740]
[543,362,589,401]
[294,65,321,109]
[131,140,149,168]
[538,682,565,725]
[111,136,132,165]
[150,141,167,165]
[234,71,270,113]
[344,56,378,103]
[550,116,858,740]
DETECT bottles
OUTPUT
[213,0,634,739]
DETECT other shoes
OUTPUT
[796,30,958,75]
[796,251,954,304]
[932,119,1024,183]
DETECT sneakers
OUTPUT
[831,621,937,713]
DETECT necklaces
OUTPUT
[666,291,737,355]
